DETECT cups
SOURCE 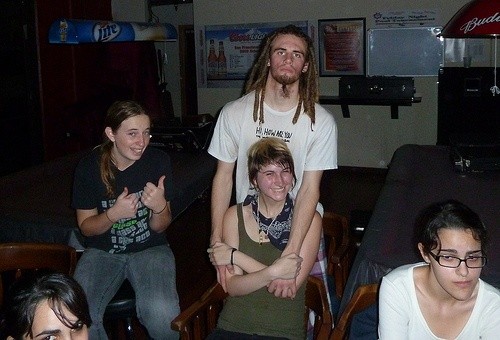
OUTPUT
[463,57,471,68]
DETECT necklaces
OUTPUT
[257,196,284,240]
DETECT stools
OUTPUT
[105,278,144,340]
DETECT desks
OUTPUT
[342,143,500,340]
[0,143,222,250]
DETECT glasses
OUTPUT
[427,242,487,269]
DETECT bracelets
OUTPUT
[231,248,237,265]
[154,202,167,214]
[106,209,113,223]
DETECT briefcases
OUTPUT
[339,76,416,101]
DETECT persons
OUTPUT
[72,97,182,340]
[0,269,93,340]
[378,200,500,340]
[205,23,338,339]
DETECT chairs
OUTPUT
[170,212,379,339]
[0,237,76,308]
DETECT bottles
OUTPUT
[217,41,227,80]
[208,39,218,80]
